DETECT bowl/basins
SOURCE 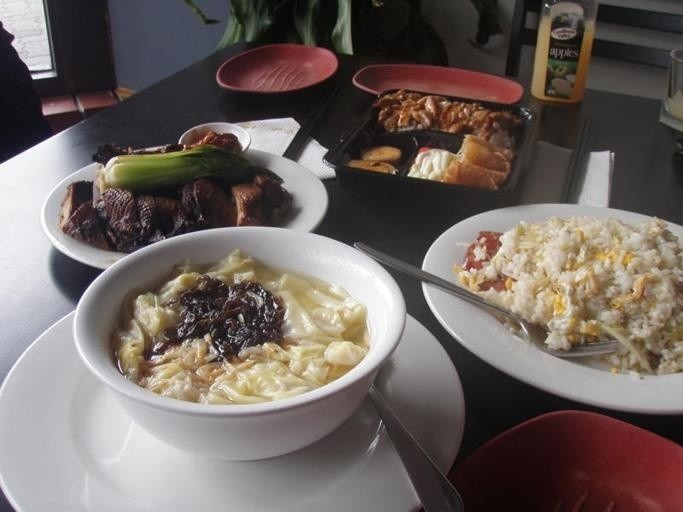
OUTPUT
[455,411,677,512]
[70,225,402,462]
[323,88,543,204]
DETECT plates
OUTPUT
[352,65,523,104]
[38,145,330,273]
[0,309,468,510]
[216,43,339,99]
[179,119,251,153]
[420,205,683,414]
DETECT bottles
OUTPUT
[530,0,601,105]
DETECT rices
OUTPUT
[456,213,683,373]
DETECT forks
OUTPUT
[359,242,623,360]
